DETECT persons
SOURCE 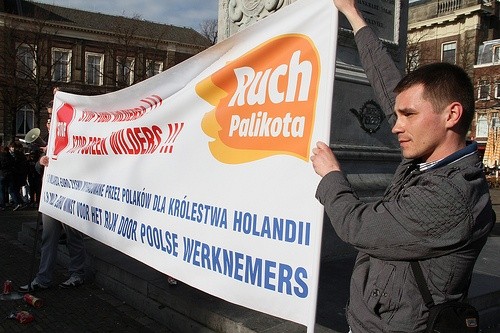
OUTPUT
[1,116,53,209]
[20,87,86,290]
[310,0,497,333]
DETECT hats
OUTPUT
[7,141,17,147]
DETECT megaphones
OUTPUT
[26,128,47,147]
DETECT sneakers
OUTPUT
[17,282,47,293]
[59,275,86,289]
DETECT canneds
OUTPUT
[23,293,40,306]
[3,280,11,295]
